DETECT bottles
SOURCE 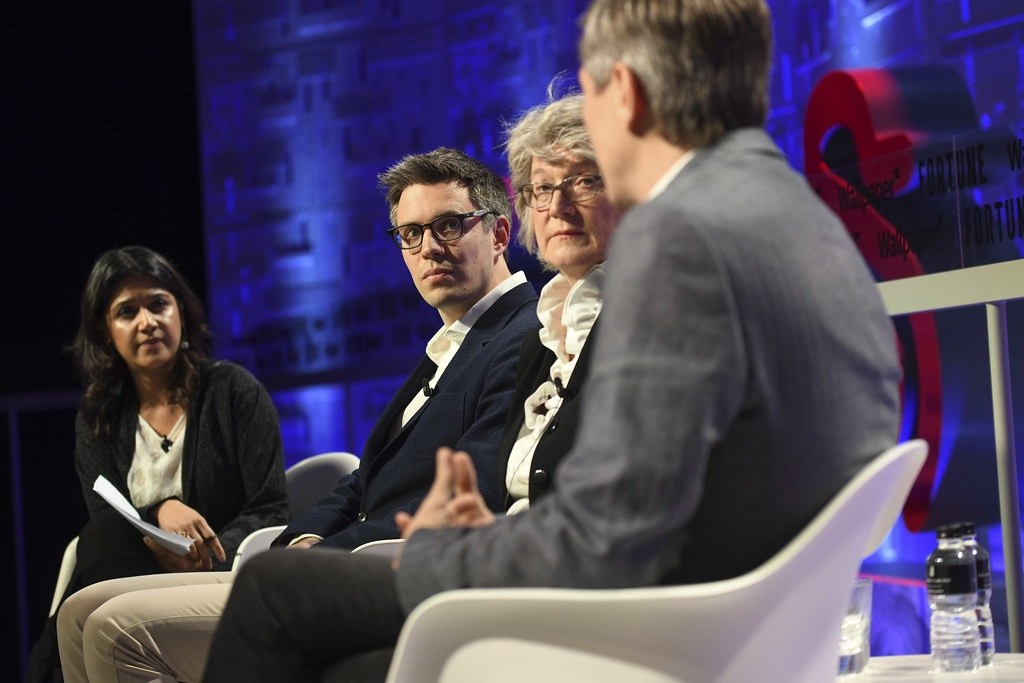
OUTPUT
[922,523,981,676]
[956,521,995,666]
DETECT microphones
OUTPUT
[161,440,172,452]
[422,378,433,396]
[555,377,566,397]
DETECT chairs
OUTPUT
[384,441,926,682]
[47,450,362,621]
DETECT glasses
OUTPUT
[386,208,500,249]
[518,173,605,210]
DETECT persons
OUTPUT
[23,246,288,683]
[485,91,627,517]
[55,145,544,683]
[196,0,903,682]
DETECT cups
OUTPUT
[837,576,875,675]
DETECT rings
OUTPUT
[177,530,189,539]
